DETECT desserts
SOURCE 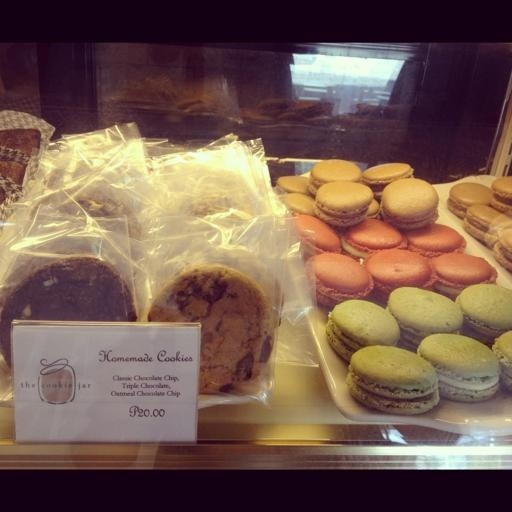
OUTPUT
[275,127,512,417]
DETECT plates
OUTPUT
[278,180,511,437]
[2,370,274,411]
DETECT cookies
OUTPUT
[0,200,281,395]
